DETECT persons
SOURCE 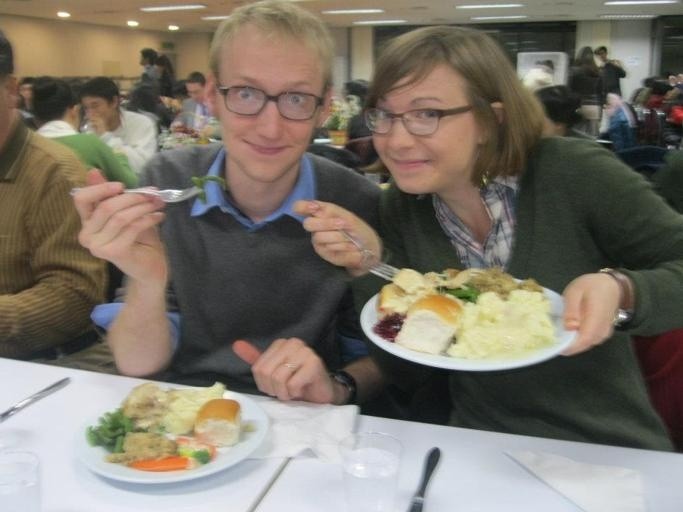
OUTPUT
[73,0,383,404]
[292,21,682,453]
[525,45,683,165]
[17,48,214,188]
[1,29,111,358]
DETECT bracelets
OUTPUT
[330,369,359,405]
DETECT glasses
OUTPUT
[361,104,473,137]
[216,79,324,122]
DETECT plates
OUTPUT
[359,278,577,376]
[71,388,271,487]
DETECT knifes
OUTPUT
[0,377,72,425]
[409,446,439,512]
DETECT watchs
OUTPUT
[600,265,633,329]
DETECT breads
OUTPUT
[392,294,465,355]
[192,398,242,448]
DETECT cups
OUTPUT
[79,119,96,134]
[336,432,403,510]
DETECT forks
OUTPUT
[68,182,198,205]
[336,229,401,284]
